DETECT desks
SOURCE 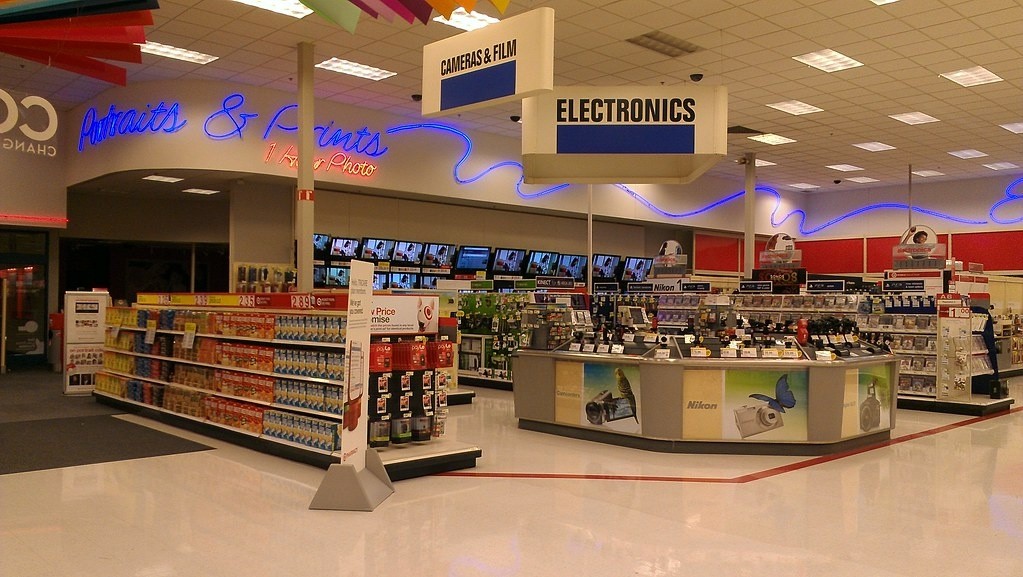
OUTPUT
[510,348,902,458]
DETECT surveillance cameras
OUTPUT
[511,116,520,122]
[834,180,841,184]
[690,74,704,82]
[412,95,422,101]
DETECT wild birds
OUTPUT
[613,367,641,425]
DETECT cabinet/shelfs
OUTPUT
[98,281,1023,486]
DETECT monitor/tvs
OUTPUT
[311,232,654,296]
[618,306,648,328]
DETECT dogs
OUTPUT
[866,377,878,395]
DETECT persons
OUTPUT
[399,275,408,289]
[537,254,550,274]
[503,252,516,271]
[632,260,643,280]
[402,243,414,261]
[599,258,612,277]
[902,226,928,259]
[372,241,384,259]
[314,234,323,249]
[566,257,579,278]
[334,270,346,285]
[430,278,437,289]
[340,241,351,256]
[433,247,447,266]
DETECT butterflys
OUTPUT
[749,373,796,414]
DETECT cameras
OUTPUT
[858,383,882,431]
[572,331,584,339]
[658,334,670,344]
[586,390,635,425]
[720,316,859,349]
[733,404,784,438]
